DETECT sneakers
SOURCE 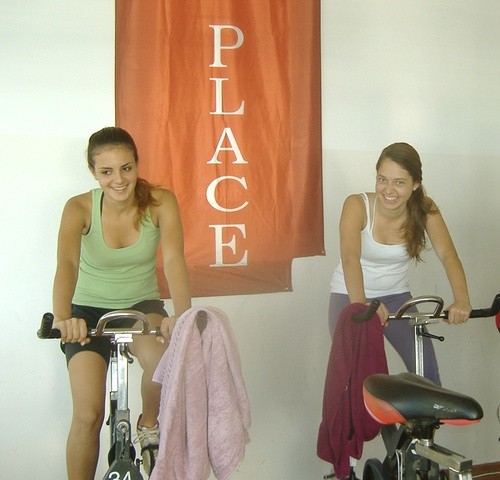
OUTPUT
[133,413,160,476]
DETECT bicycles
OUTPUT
[37,310,206,480]
[341,295,500,479]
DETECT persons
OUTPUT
[52,127,192,480]
[329,142,474,480]
